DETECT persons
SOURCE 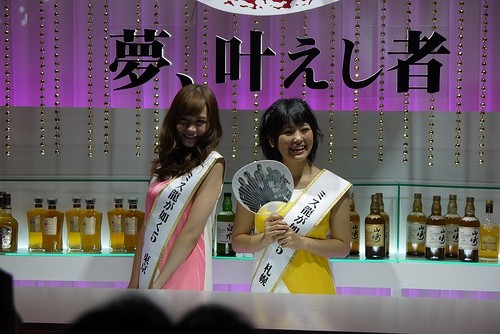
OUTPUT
[126,83,227,291]
[232,98,351,295]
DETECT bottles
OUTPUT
[0,194,18,252]
[424,195,445,260]
[123,197,145,253]
[216,190,236,255]
[375,192,390,256]
[458,197,480,262]
[25,196,46,251]
[405,193,426,256]
[40,198,65,252]
[478,199,500,260]
[107,196,125,253]
[364,195,385,259]
[349,193,360,257]
[79,197,103,254]
[0,191,6,215]
[65,197,83,251]
[445,194,462,259]
